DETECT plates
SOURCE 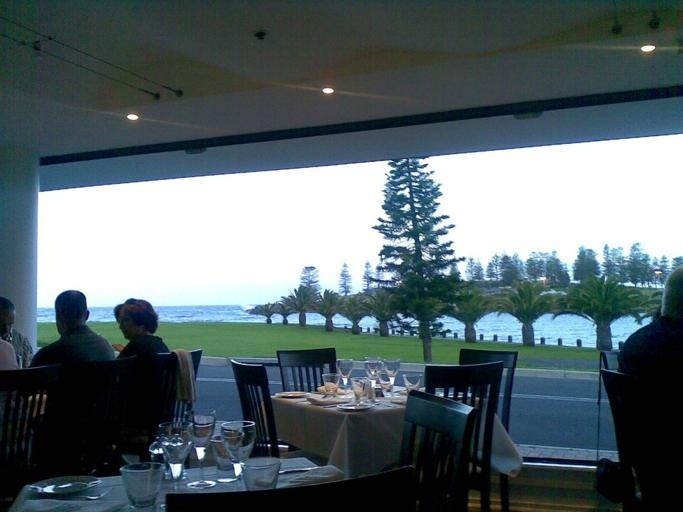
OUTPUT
[27,475,102,497]
[274,392,310,398]
[337,403,374,411]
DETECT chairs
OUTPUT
[0,365,61,498]
[276,346,337,392]
[415,361,505,512]
[225,357,328,466]
[83,355,141,476]
[121,349,203,463]
[458,349,519,512]
[601,369,657,512]
[379,389,477,512]
[165,466,417,512]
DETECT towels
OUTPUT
[294,465,346,484]
[309,395,349,405]
[21,500,126,512]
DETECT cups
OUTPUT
[240,456,283,490]
[158,421,196,492]
[322,373,340,399]
[221,420,257,491]
[382,359,401,395]
[364,355,384,403]
[120,462,166,510]
[335,358,354,386]
[349,377,367,405]
[182,408,218,490]
[403,373,423,393]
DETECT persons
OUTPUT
[114,299,171,463]
[619,270,683,512]
[1,298,36,369]
[30,290,117,476]
[0,337,36,456]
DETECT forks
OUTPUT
[59,487,114,502]
